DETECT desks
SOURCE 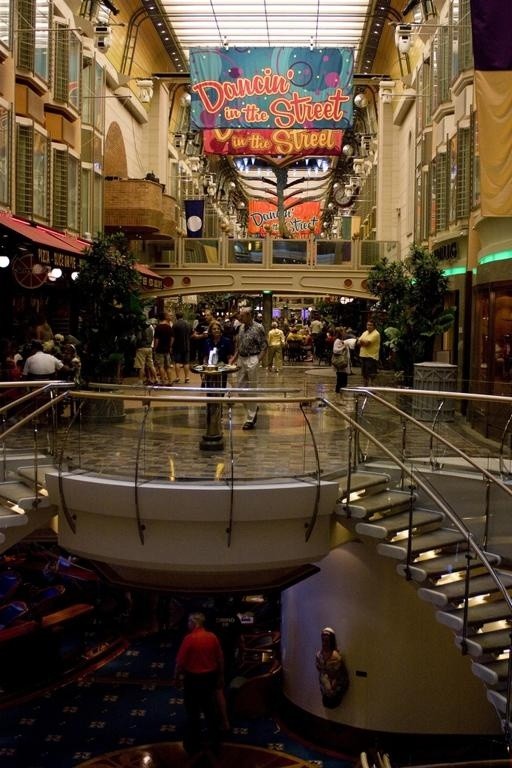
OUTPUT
[189,362,242,451]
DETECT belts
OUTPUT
[240,353,256,357]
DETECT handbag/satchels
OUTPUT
[280,337,286,347]
[331,354,348,368]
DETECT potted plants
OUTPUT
[68,232,152,422]
[365,242,459,387]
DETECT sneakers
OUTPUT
[143,377,191,386]
[242,405,260,429]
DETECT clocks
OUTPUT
[333,186,357,207]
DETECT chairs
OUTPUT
[25,371,60,427]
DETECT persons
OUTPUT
[133,307,381,406]
[175,611,231,756]
[228,307,268,430]
[0,312,82,424]
[201,321,232,420]
[316,627,343,709]
